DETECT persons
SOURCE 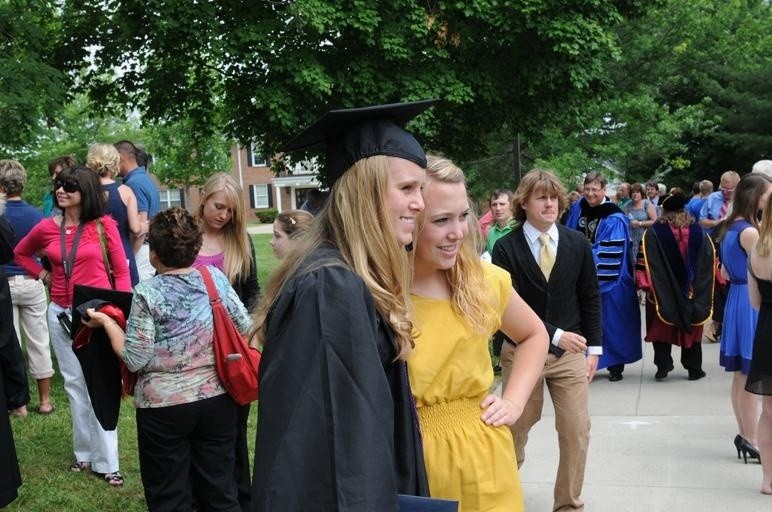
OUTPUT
[480,190,522,378]
[404,156,548,512]
[646,180,669,206]
[691,179,713,234]
[753,160,772,179]
[80,206,258,512]
[685,182,701,211]
[623,183,658,304]
[44,155,79,218]
[271,210,318,259]
[618,182,633,212]
[492,168,604,512]
[745,195,772,494]
[0,267,31,509]
[477,211,495,239]
[614,192,621,205]
[657,183,666,196]
[13,166,132,487]
[641,184,647,198]
[86,142,150,287]
[704,201,731,343]
[115,140,162,283]
[634,187,715,381]
[701,171,742,237]
[721,174,761,465]
[0,157,56,414]
[159,172,258,512]
[576,185,584,192]
[560,191,581,226]
[136,147,147,168]
[560,171,642,381]
[252,99,458,511]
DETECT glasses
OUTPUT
[584,188,602,191]
[718,182,735,192]
[54,179,81,195]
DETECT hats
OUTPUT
[279,97,443,188]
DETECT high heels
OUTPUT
[732,434,744,460]
[739,437,762,465]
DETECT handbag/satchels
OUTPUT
[195,261,264,406]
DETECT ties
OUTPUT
[537,232,556,281]
[718,199,731,219]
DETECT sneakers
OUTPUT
[652,368,669,380]
[687,368,706,381]
[607,372,623,381]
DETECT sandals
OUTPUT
[67,460,89,474]
[38,403,56,416]
[103,471,124,488]
